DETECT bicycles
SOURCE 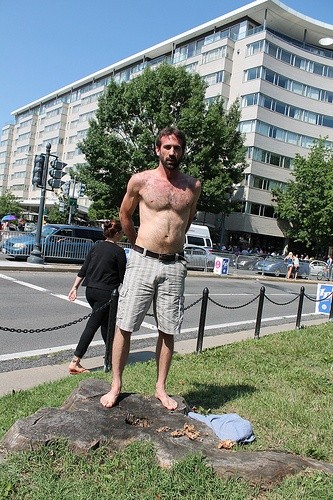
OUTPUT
[317,266,333,281]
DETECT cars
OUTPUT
[183,244,222,271]
[239,255,333,277]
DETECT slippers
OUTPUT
[69,366,86,374]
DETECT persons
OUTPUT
[216,243,333,282]
[68,219,127,374]
[100,126,200,410]
[0,218,37,234]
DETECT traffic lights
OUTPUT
[48,160,68,189]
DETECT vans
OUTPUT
[185,223,214,251]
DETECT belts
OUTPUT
[133,245,189,263]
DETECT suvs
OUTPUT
[0,223,107,265]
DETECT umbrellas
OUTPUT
[1,215,17,225]
